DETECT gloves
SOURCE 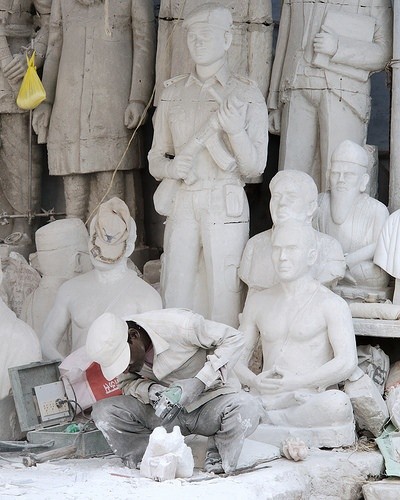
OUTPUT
[169,378,204,409]
[149,384,168,401]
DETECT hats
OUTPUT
[86,313,130,381]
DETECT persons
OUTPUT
[0,269,40,401]
[18,218,94,352]
[314,139,392,292]
[32,0,156,233]
[237,169,348,290]
[234,220,359,427]
[266,0,393,194]
[152,0,273,103]
[0,0,53,245]
[85,306,266,476]
[146,3,268,330]
[41,198,164,365]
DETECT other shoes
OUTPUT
[204,447,223,474]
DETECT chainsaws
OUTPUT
[152,386,182,426]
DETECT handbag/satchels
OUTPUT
[58,344,124,415]
[16,49,46,110]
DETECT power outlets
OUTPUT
[43,397,69,417]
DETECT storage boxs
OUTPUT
[8,359,114,458]
